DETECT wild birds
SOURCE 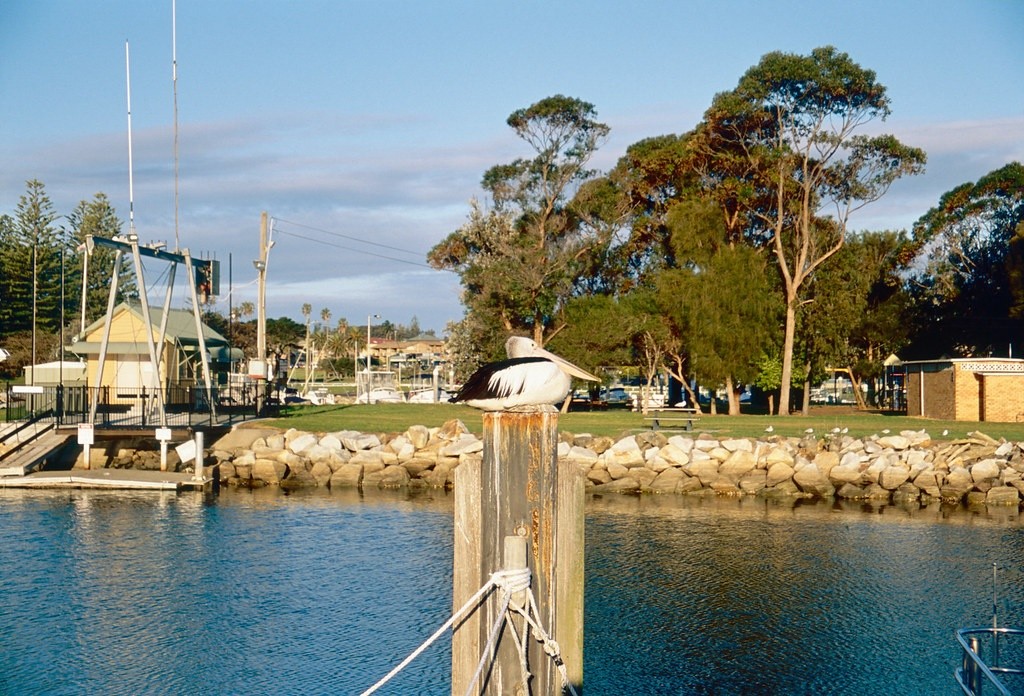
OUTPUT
[762,423,949,440]
[447,335,602,413]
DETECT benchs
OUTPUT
[643,417,701,432]
[645,407,697,418]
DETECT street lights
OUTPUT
[368,312,381,403]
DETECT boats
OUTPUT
[407,390,452,402]
[354,387,402,403]
